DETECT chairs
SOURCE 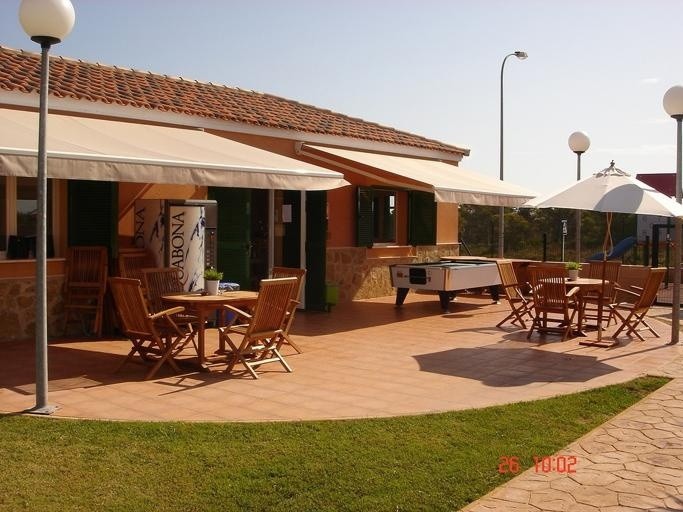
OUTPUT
[60,246,308,380]
[494,260,668,348]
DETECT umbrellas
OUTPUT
[518,160,683,343]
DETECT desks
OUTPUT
[389,260,508,314]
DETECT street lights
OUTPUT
[498,51,529,181]
[662,84,683,343]
[17,0,76,406]
[567,131,590,264]
